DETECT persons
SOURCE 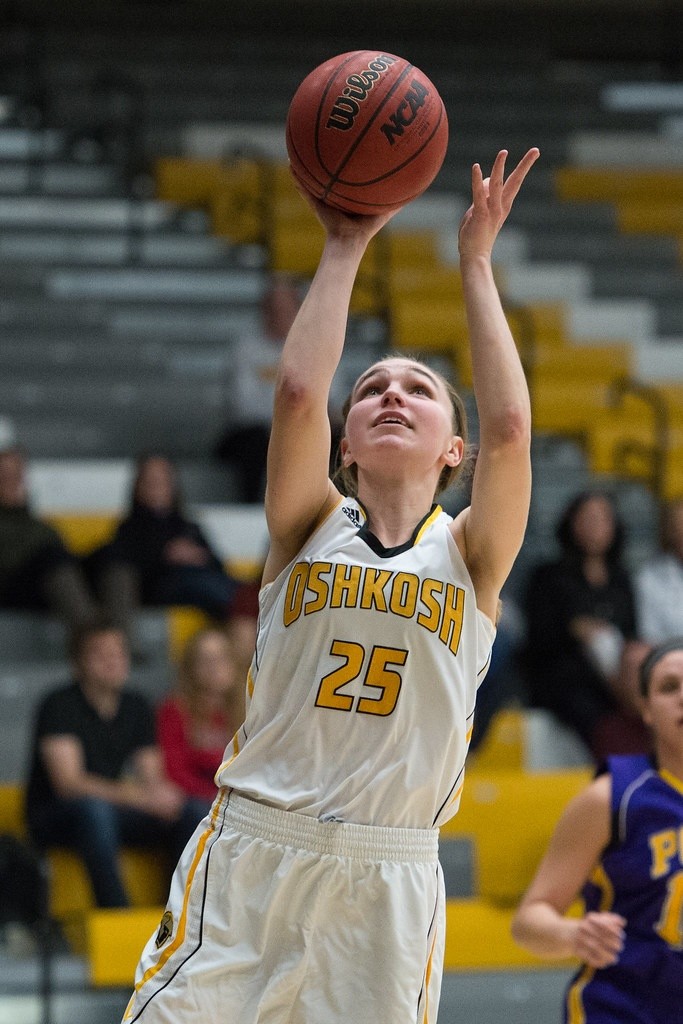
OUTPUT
[121,149,543,1024]
[0,445,683,910]
[510,637,683,1024]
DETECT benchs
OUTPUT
[0,73,681,991]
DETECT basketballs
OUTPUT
[283,50,450,216]
